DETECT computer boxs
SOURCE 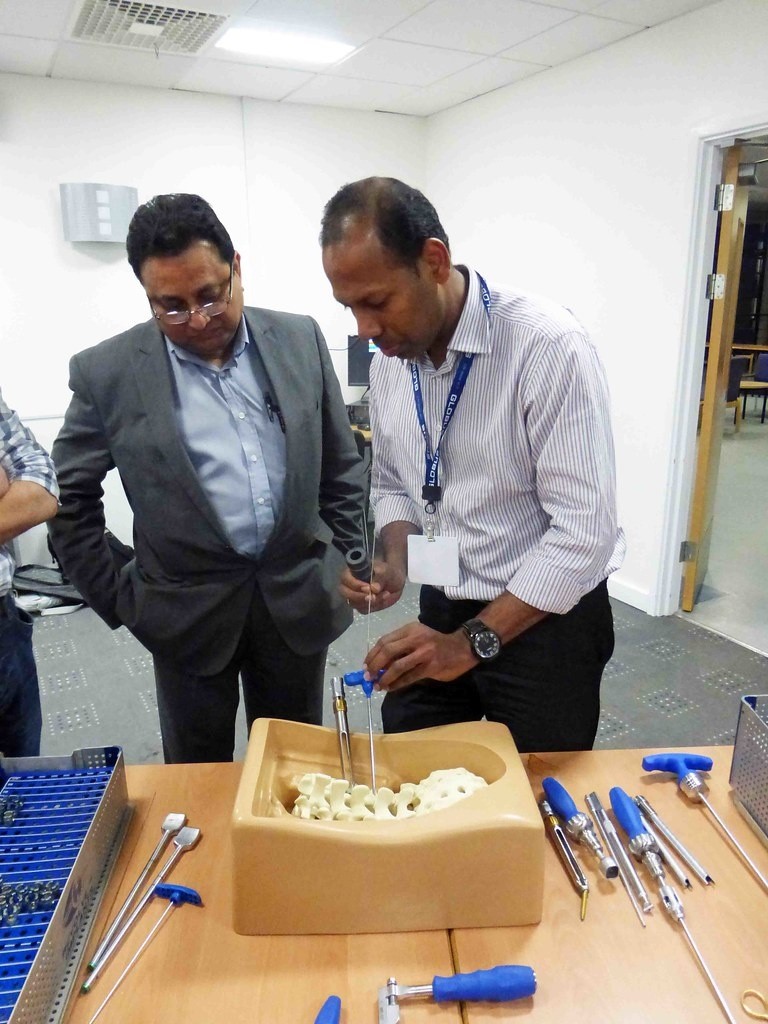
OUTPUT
[344,401,371,425]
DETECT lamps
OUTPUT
[60,182,139,243]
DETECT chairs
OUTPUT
[696,353,768,433]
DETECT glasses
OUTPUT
[148,262,234,324]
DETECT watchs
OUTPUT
[462,620,502,663]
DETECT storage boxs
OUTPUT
[729,693,768,837]
[0,745,129,1024]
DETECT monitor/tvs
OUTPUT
[347,335,377,401]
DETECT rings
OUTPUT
[346,598,350,604]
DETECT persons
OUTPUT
[318,177,621,753]
[0,387,60,758]
[47,194,366,766]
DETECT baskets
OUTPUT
[728,694,768,835]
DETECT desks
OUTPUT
[69,744,768,1024]
[706,343,768,353]
